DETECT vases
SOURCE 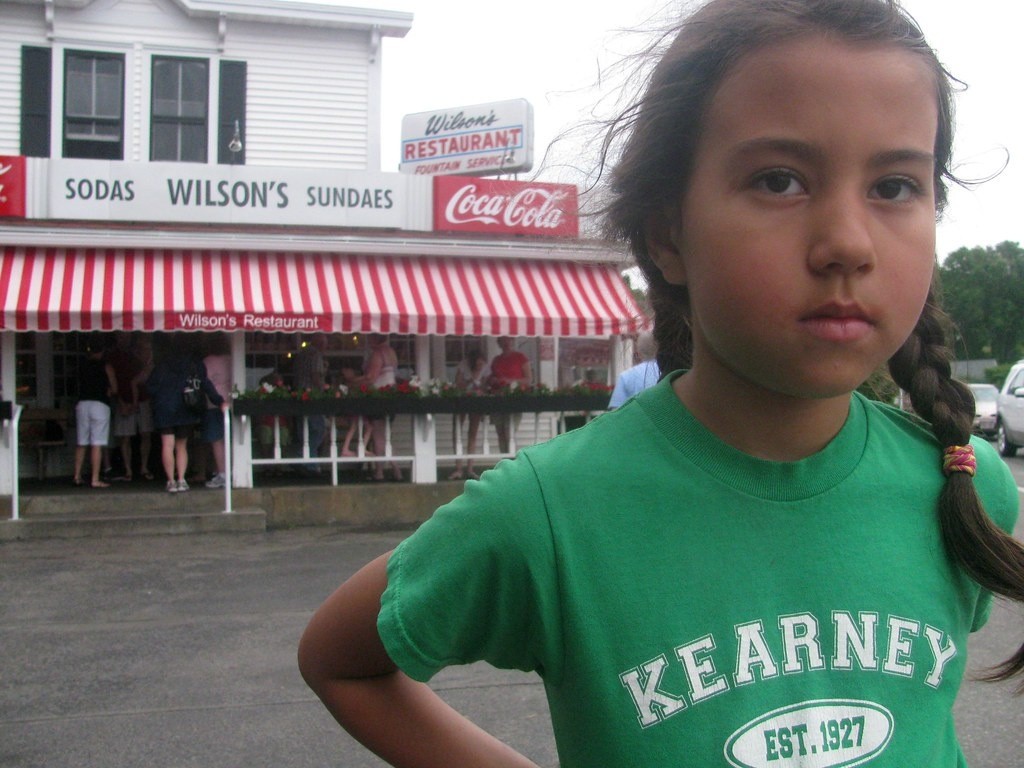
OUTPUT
[235,394,617,413]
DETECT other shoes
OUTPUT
[205,472,226,488]
[73,479,84,489]
[91,481,112,489]
[340,450,482,482]
[176,480,190,491]
[165,481,177,492]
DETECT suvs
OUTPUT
[997,360,1023,458]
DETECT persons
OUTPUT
[483,334,531,453]
[446,347,492,481]
[361,332,407,484]
[573,361,606,387]
[115,333,155,483]
[198,330,230,490]
[134,333,228,494]
[607,357,665,412]
[336,359,375,459]
[67,341,121,488]
[275,333,328,477]
[296,1,1022,766]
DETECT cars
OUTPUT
[966,383,1000,437]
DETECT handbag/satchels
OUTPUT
[182,356,208,417]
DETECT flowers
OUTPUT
[227,373,616,399]
